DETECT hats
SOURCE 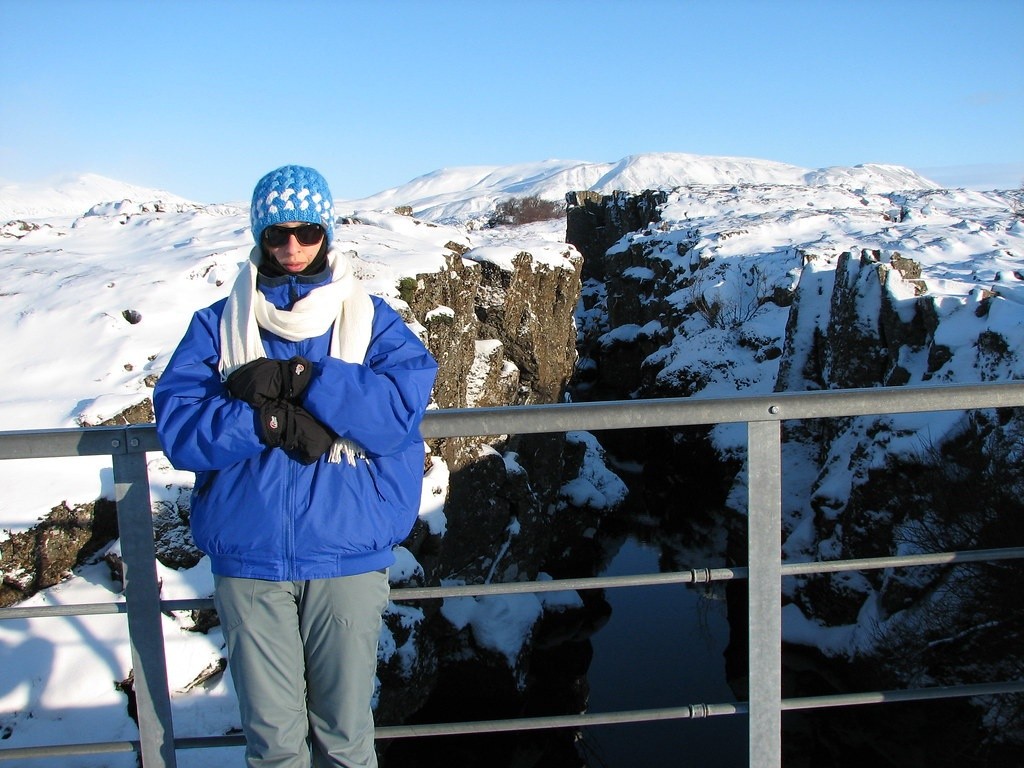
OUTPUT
[250,164,334,253]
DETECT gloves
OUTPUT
[257,399,333,466]
[228,355,313,408]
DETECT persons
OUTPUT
[154,166,439,768]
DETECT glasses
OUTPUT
[263,222,324,249]
